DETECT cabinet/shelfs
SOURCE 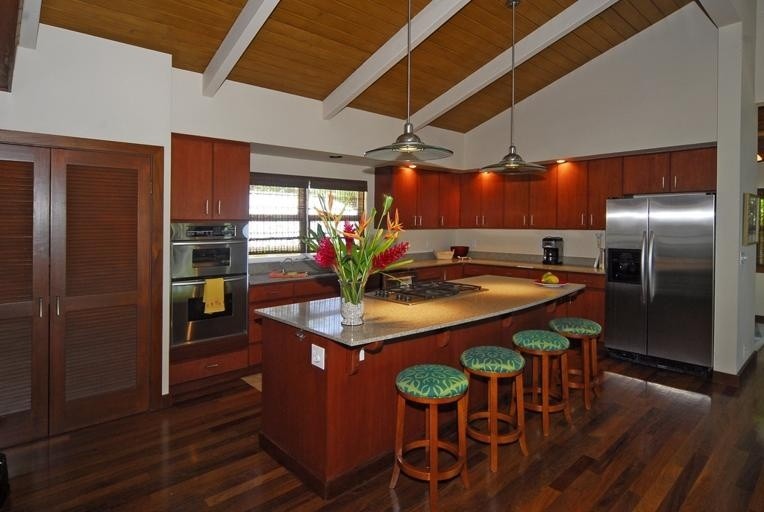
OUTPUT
[170,132,252,224]
[372,165,438,231]
[438,172,460,231]
[245,260,610,367]
[169,344,248,406]
[556,156,622,231]
[622,145,716,196]
[501,161,556,231]
[460,169,501,231]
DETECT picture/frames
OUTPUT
[741,191,760,248]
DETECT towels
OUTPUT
[201,278,226,316]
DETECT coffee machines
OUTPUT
[542,237,564,265]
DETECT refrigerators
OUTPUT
[602,191,715,380]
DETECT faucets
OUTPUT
[279,257,294,275]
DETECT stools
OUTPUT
[505,328,576,439]
[546,316,602,412]
[455,343,530,475]
[379,363,472,512]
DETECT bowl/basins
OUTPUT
[450,246,470,259]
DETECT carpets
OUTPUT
[238,372,263,394]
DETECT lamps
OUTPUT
[359,0,456,166]
[476,1,551,177]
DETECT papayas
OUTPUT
[541,272,560,284]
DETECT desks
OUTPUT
[251,273,590,505]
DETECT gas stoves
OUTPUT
[362,279,482,307]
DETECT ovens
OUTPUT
[170,220,250,349]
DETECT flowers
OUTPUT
[294,185,416,303]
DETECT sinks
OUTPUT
[268,271,312,278]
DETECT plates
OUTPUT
[533,280,570,288]
